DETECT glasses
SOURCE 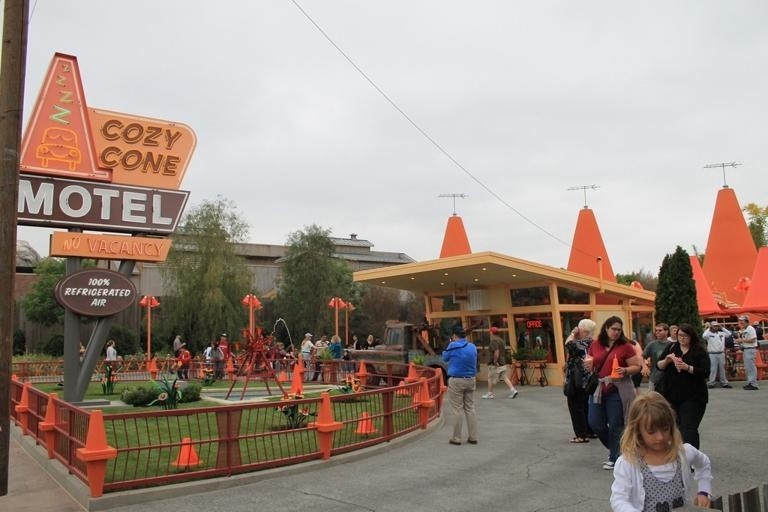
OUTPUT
[611,326,622,332]
[677,333,690,339]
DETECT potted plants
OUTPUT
[319,344,345,384]
[512,345,530,387]
[529,344,550,388]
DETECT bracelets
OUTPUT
[625,368,627,375]
[697,491,708,496]
[571,332,575,336]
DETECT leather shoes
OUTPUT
[743,384,759,390]
[721,385,733,388]
[708,385,714,388]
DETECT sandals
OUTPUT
[571,435,589,443]
[588,434,598,439]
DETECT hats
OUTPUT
[489,327,498,334]
[305,333,312,337]
[738,315,749,321]
[451,327,465,335]
[710,321,719,326]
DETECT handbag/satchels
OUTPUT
[563,373,587,396]
[585,374,599,396]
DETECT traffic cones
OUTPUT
[172,438,206,466]
[610,359,625,379]
[396,378,411,396]
[412,389,419,410]
[351,411,377,434]
[288,365,304,394]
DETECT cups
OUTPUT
[582,355,594,371]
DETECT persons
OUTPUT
[442,327,477,445]
[481,326,518,400]
[218,333,229,361]
[203,342,213,365]
[105,339,117,381]
[175,345,192,380]
[581,316,643,469]
[627,314,765,473]
[299,332,379,381]
[609,390,714,512]
[565,318,597,444]
[169,335,186,374]
[209,341,224,380]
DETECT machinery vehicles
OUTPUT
[351,321,482,390]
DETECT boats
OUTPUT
[277,370,286,383]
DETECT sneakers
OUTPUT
[468,438,477,444]
[482,394,494,399]
[450,438,461,445]
[508,390,518,399]
[603,461,614,470]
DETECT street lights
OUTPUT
[327,297,346,340]
[241,293,261,340]
[342,300,355,346]
[139,294,160,369]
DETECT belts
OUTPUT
[303,351,310,353]
[708,352,723,354]
[451,376,473,379]
